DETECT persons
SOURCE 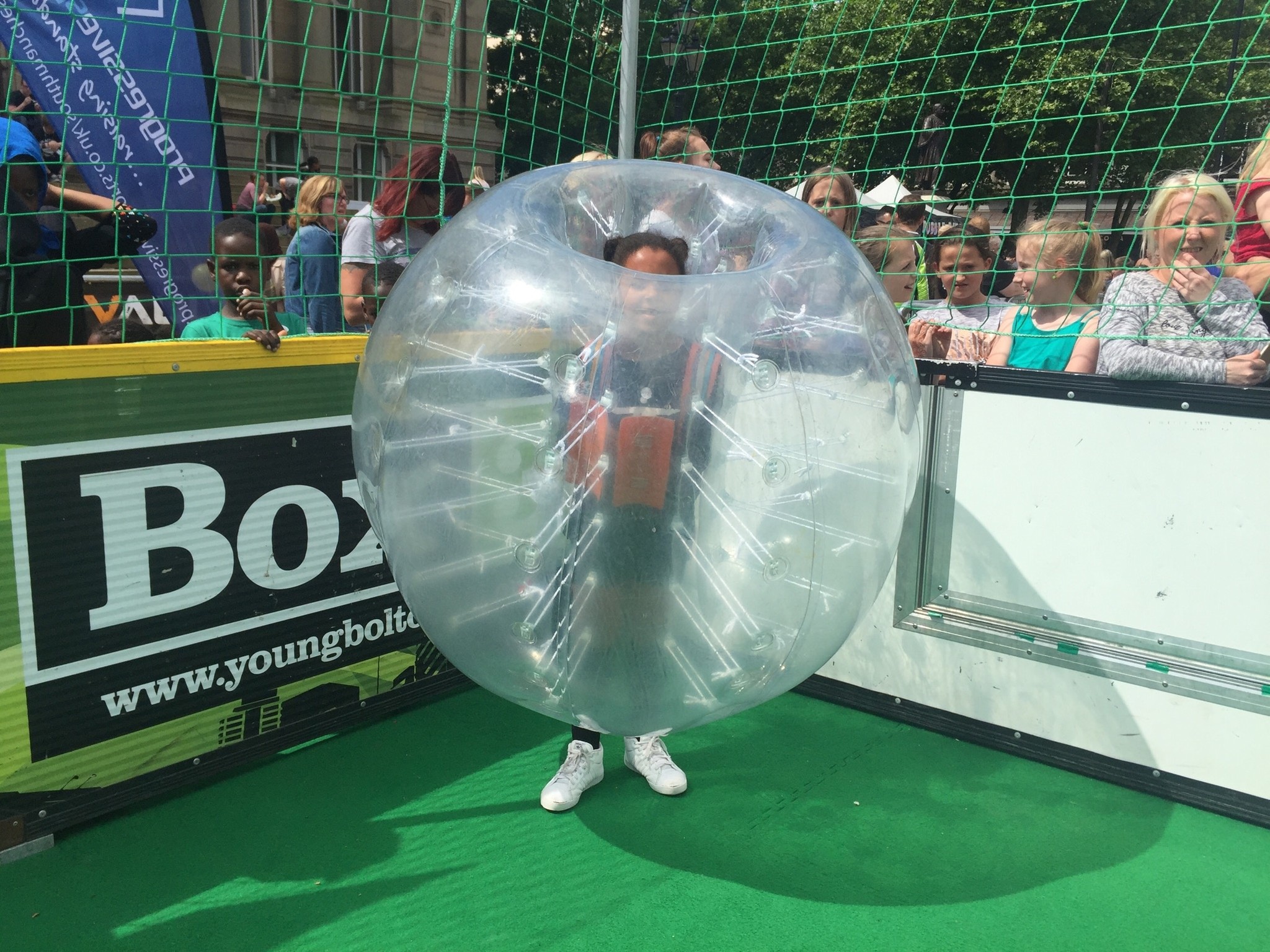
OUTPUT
[539,234,724,814]
[0,131,1269,391]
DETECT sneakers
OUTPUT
[540,740,604,811]
[623,728,686,796]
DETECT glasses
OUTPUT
[419,192,440,215]
[321,193,350,204]
